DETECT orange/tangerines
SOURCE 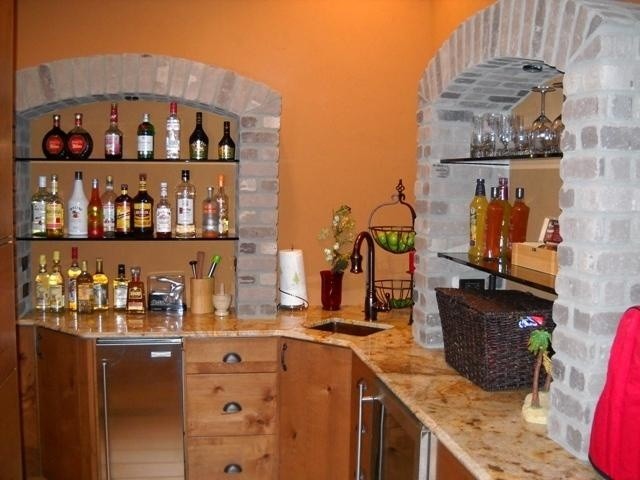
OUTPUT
[376,231,416,251]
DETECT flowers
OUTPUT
[317,204,358,272]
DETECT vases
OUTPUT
[319,270,345,311]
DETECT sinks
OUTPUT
[302,317,397,339]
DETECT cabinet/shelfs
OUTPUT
[183,337,354,480]
[438,151,563,295]
[36,327,101,479]
[15,158,239,242]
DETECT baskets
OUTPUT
[370,279,415,309]
[434,287,552,393]
[370,225,417,254]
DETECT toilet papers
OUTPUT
[277,248,307,307]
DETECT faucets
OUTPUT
[350,229,393,321]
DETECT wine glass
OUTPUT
[529,81,565,154]
[212,293,232,316]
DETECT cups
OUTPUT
[469,105,530,158]
[189,276,215,314]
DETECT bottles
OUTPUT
[29,99,236,238]
[469,175,531,262]
[35,245,146,314]
